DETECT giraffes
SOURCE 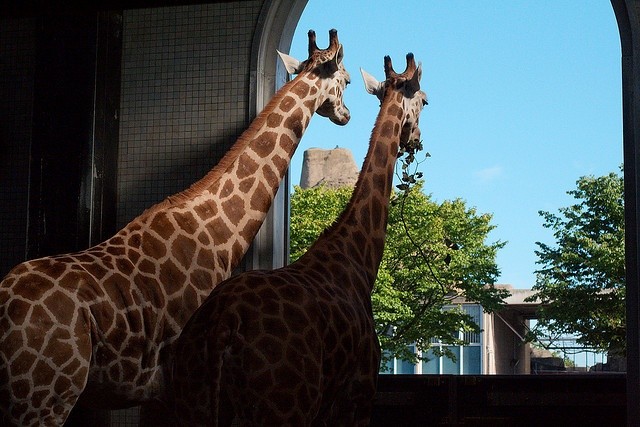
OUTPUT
[1,29,353,426]
[172,52,430,426]
